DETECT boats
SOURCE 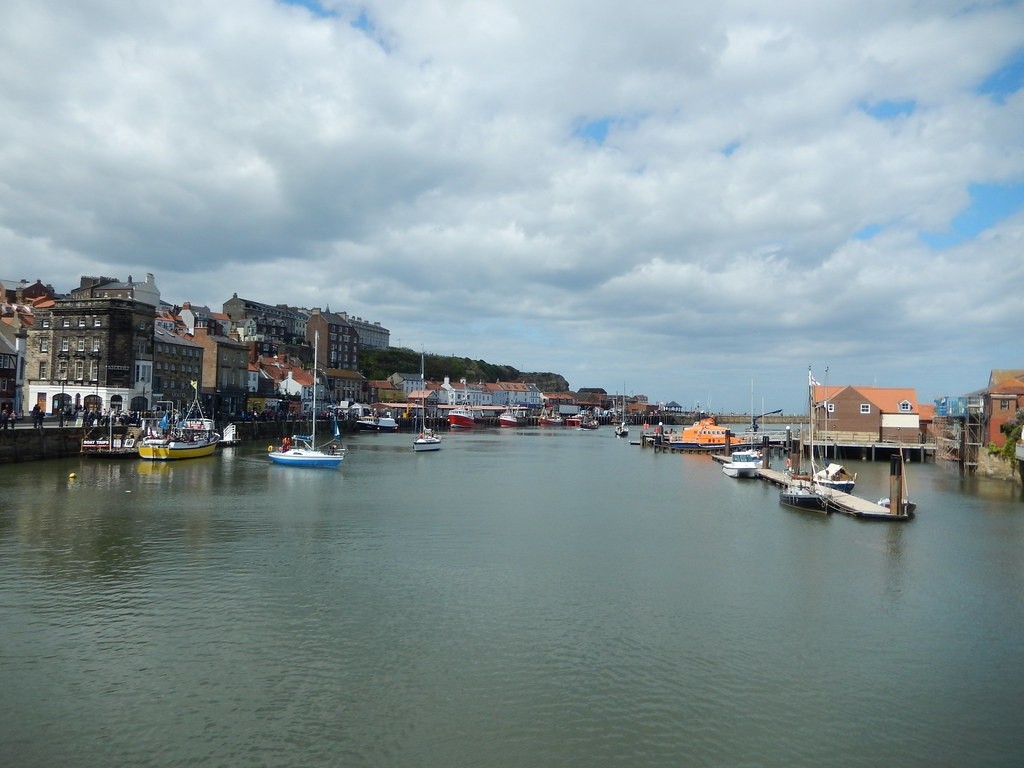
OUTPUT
[76,409,141,457]
[498,398,519,427]
[445,380,476,428]
[813,365,857,492]
[358,415,399,433]
[613,378,628,437]
[266,330,347,470]
[721,449,760,480]
[668,416,747,452]
[778,477,829,514]
[139,379,220,461]
[578,412,599,429]
[538,401,562,426]
[409,342,442,451]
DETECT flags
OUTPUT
[190,380,197,389]
[812,376,820,386]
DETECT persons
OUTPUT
[787,456,791,470]
[0,400,384,429]
